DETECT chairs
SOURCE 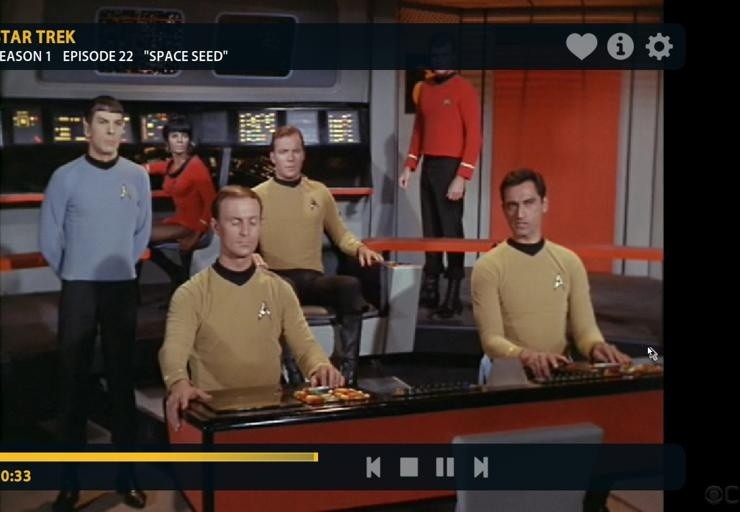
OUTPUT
[148,147,232,296]
[276,244,378,332]
[447,421,608,510]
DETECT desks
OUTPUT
[3,185,375,205]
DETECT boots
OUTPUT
[420,279,470,316]
[341,314,368,388]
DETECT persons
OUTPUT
[250,126,385,390]
[37,96,152,512]
[397,69,484,320]
[471,169,632,388]
[158,184,346,433]
[140,114,220,288]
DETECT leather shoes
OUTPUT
[117,487,148,509]
[59,488,83,508]
